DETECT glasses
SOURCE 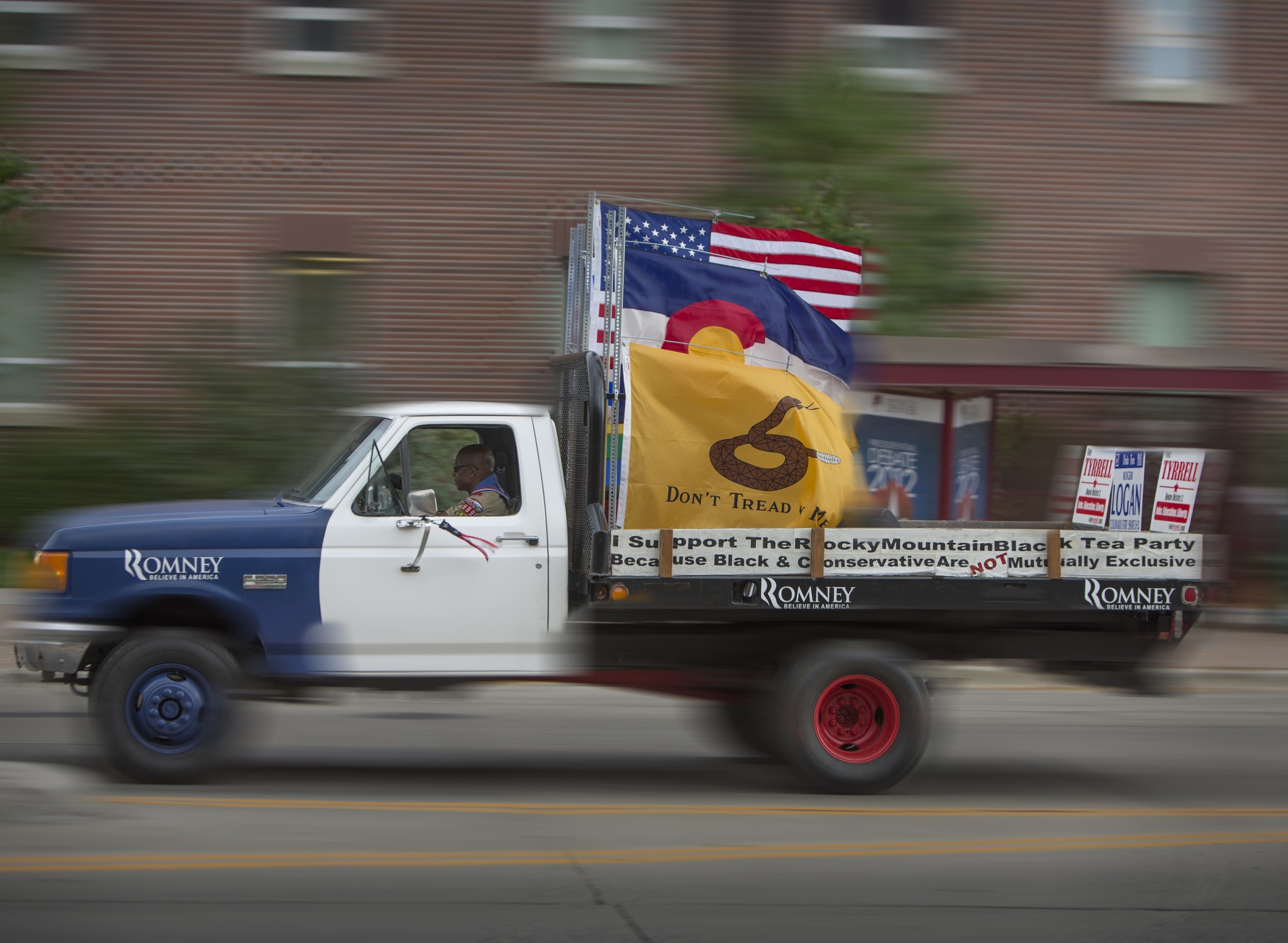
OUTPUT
[453,464,480,472]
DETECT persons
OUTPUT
[435,443,510,516]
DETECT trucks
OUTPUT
[4,190,1206,798]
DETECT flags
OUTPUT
[589,200,864,528]
